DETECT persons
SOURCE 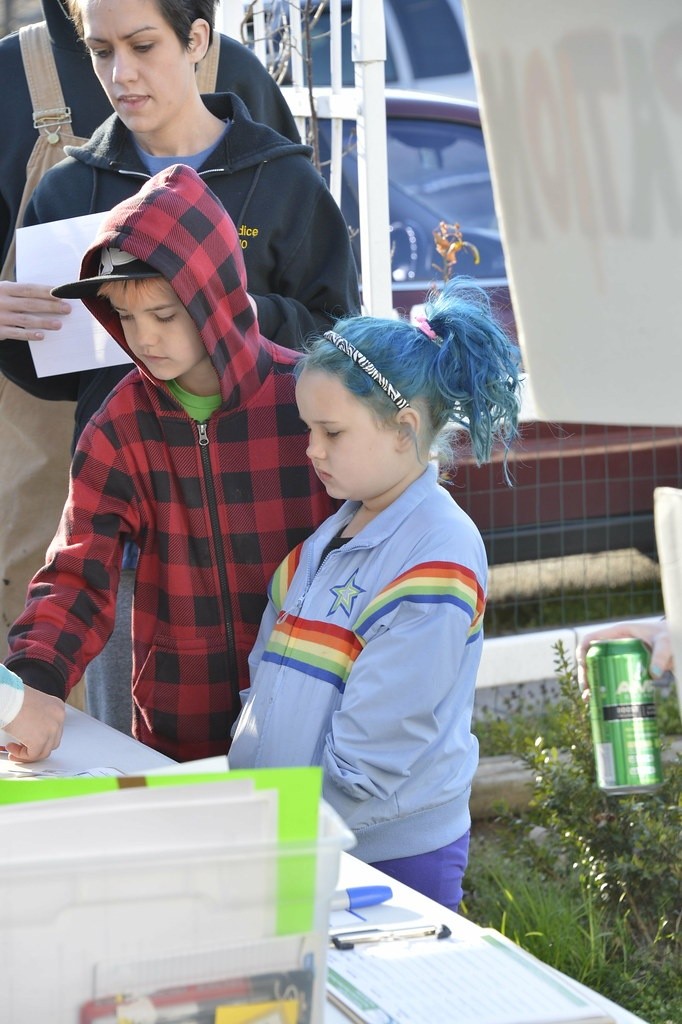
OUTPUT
[223,273,525,920]
[0,0,367,747]
[0,664,66,762]
[0,0,297,680]
[1,167,348,769]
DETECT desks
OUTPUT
[0,704,654,1024]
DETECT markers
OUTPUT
[334,886,392,912]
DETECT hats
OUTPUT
[49,244,162,299]
